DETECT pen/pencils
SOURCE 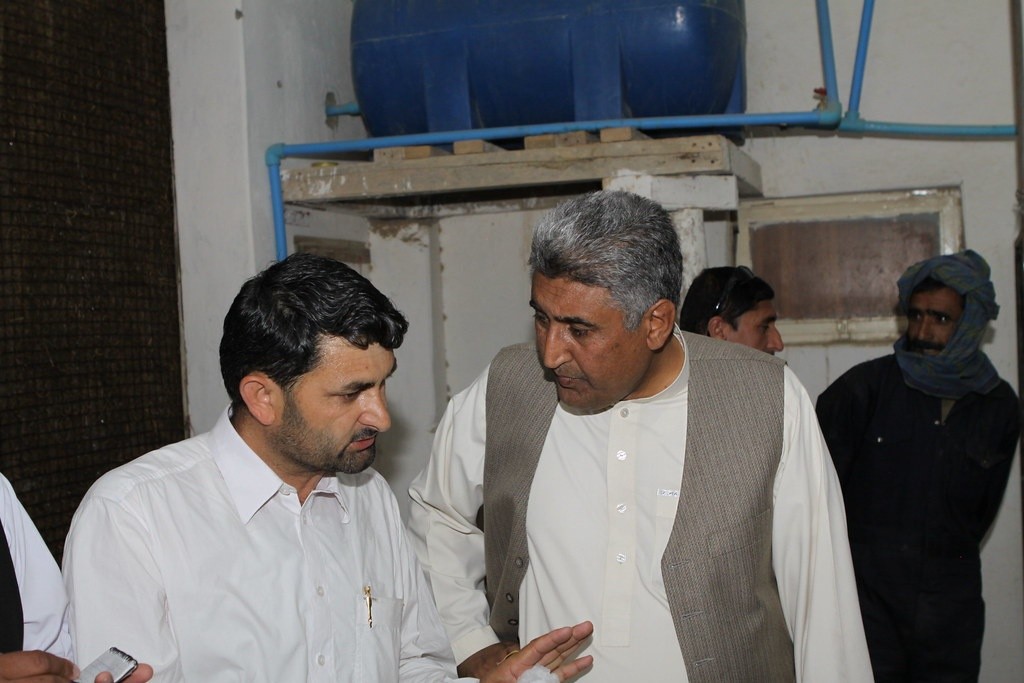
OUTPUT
[364,585,373,629]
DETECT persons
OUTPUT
[0,473,154,683]
[59,251,594,683]
[814,248,1024,683]
[679,265,785,357]
[407,188,873,682]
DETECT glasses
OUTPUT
[705,265,755,322]
